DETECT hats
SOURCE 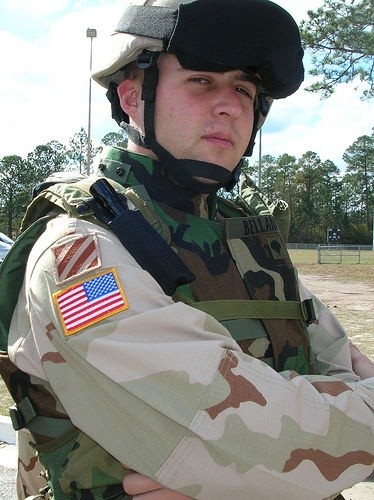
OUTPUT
[90,0,305,133]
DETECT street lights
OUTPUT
[86,28,97,177]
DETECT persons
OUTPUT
[0,0,374,500]
